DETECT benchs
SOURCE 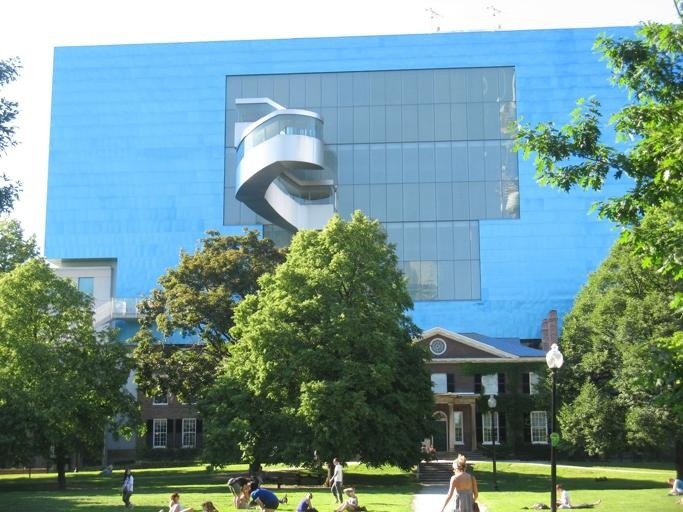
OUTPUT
[272,474,326,490]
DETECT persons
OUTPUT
[169,458,358,512]
[668,477,683,496]
[556,484,571,509]
[440,455,478,512]
[122,468,134,508]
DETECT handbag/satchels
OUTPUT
[473,502,479,512]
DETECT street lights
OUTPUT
[545,343,564,512]
[487,393,501,490]
